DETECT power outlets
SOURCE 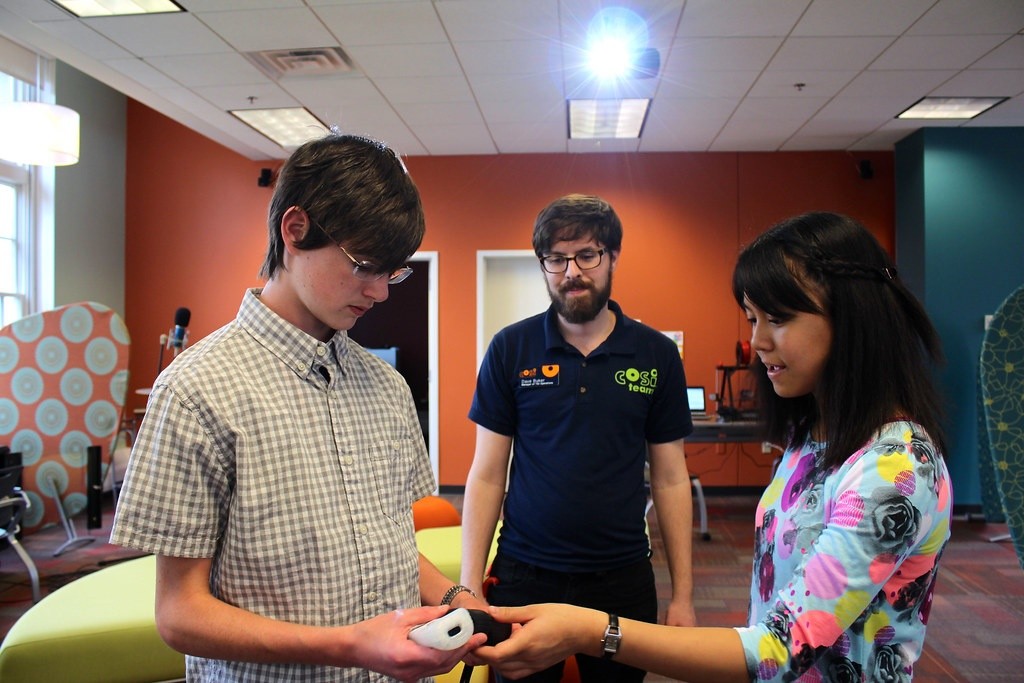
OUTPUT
[762,443,773,454]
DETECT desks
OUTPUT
[684,419,759,443]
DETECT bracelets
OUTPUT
[441,585,476,607]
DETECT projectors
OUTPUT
[631,49,661,82]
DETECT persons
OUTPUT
[109,130,491,683]
[466,210,954,683]
[458,192,694,683]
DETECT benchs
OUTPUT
[0,493,503,683]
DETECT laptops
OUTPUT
[687,386,711,420]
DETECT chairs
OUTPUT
[643,438,711,556]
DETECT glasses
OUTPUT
[309,216,413,285]
[538,247,609,274]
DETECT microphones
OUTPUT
[173,308,191,357]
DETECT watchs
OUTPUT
[601,613,622,661]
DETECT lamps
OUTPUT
[625,47,659,80]
[0,52,80,166]
